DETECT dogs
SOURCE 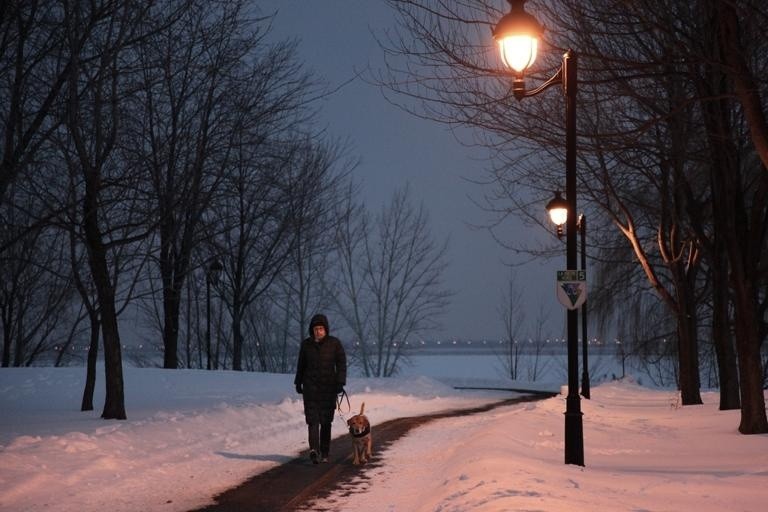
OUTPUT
[347,402,372,465]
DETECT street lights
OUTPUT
[487,0,592,475]
[542,181,596,409]
[614,338,628,379]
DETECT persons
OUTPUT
[294,315,346,463]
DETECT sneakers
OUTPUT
[310,449,319,464]
[320,457,328,463]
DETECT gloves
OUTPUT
[337,384,343,393]
[295,383,302,393]
